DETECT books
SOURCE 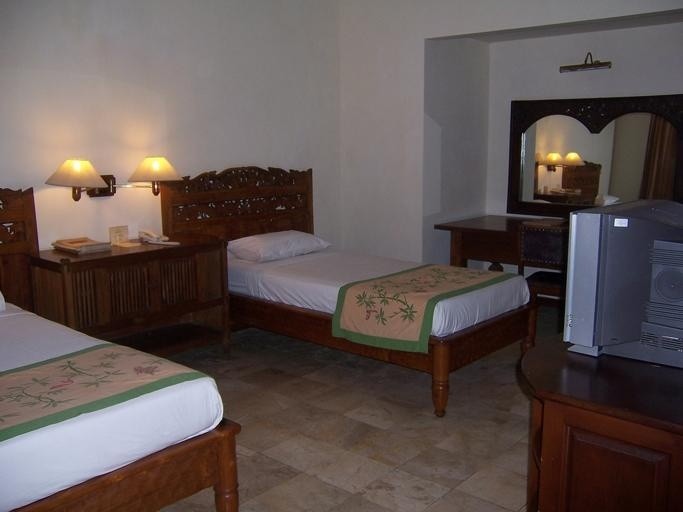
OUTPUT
[52,237,112,255]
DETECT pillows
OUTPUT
[594,193,619,206]
[227,229,331,263]
[0,291,6,311]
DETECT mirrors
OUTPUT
[506,94,682,217]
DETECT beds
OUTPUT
[0,188,242,512]
[160,166,543,417]
[562,161,624,207]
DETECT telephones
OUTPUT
[138,230,169,242]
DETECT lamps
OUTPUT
[559,50,611,74]
[111,157,185,195]
[45,159,108,200]
[536,152,587,172]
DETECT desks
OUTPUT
[434,214,570,267]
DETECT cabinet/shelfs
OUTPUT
[516,334,682,512]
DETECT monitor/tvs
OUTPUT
[563,198,683,369]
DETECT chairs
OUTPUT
[518,221,568,339]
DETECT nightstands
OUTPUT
[24,233,232,353]
